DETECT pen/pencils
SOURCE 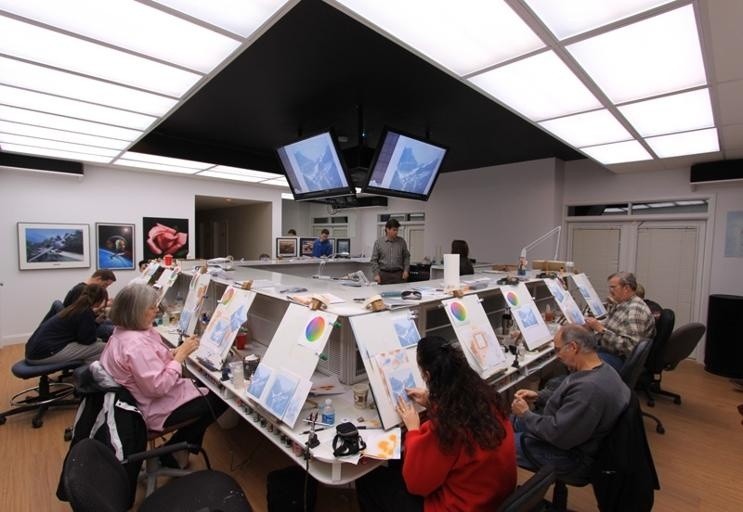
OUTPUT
[302,418,334,428]
[355,425,382,429]
[180,332,189,337]
[299,427,327,434]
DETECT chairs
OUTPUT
[497,465,557,512]
[546,336,666,433]
[77,364,211,500]
[63,440,254,512]
[654,309,675,336]
[51,299,64,316]
[513,391,661,512]
[642,322,705,407]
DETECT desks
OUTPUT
[128,257,610,486]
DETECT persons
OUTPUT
[139,260,147,272]
[584,271,658,372]
[372,219,412,285]
[451,240,474,276]
[98,283,229,470]
[286,229,296,237]
[64,270,115,343]
[511,323,631,486]
[393,336,517,512]
[312,228,332,259]
[24,284,107,400]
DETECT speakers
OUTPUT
[704,294,743,378]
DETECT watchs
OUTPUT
[602,328,606,335]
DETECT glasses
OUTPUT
[400,290,422,300]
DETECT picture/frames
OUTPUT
[337,238,350,254]
[328,238,335,254]
[96,222,136,271]
[18,222,91,270]
[298,238,319,257]
[276,236,296,257]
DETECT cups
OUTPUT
[171,311,180,324]
[444,253,460,293]
[164,254,172,265]
[567,262,574,272]
[237,333,247,350]
[200,258,207,273]
[230,361,244,389]
[242,354,259,380]
[353,384,369,407]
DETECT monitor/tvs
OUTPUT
[361,125,450,201]
[273,126,356,201]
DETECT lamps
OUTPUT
[521,225,561,260]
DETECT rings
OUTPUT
[191,342,195,346]
[401,409,405,413]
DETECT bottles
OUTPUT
[163,311,170,326]
[546,304,553,320]
[323,399,335,424]
[502,307,513,335]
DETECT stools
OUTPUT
[0,356,84,429]
